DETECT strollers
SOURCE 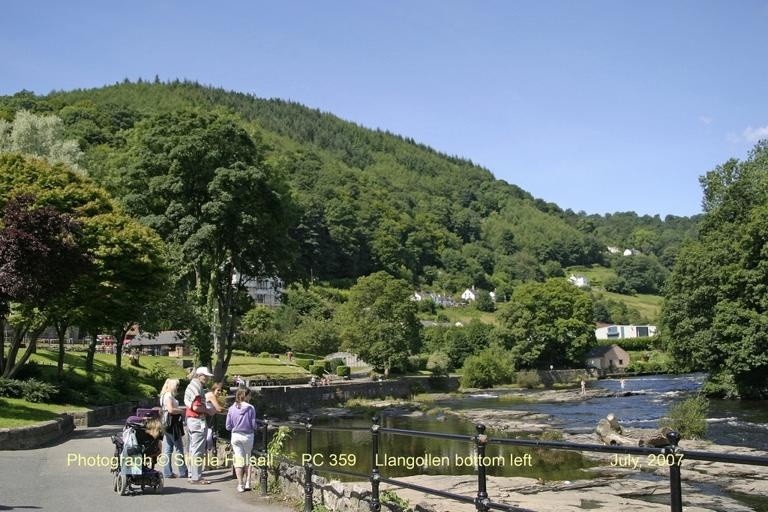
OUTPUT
[108,420,168,495]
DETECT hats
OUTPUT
[195,367,214,377]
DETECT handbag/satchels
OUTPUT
[161,411,171,429]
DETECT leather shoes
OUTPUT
[190,480,208,485]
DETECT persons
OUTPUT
[159,376,188,479]
[619,377,625,392]
[184,382,223,473]
[286,348,293,362]
[182,366,216,485]
[581,378,586,395]
[225,386,257,492]
[310,376,329,386]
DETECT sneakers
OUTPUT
[236,485,244,492]
[244,482,251,490]
